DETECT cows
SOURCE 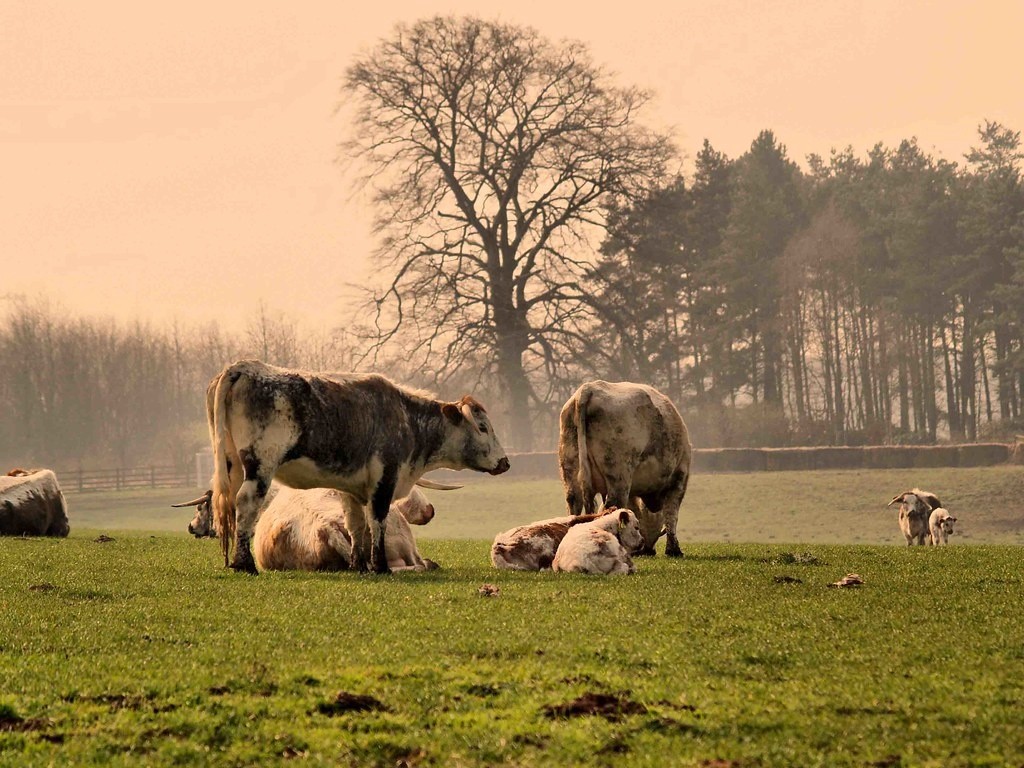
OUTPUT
[171,358,510,581]
[0,467,71,539]
[888,488,957,546]
[491,379,692,577]
